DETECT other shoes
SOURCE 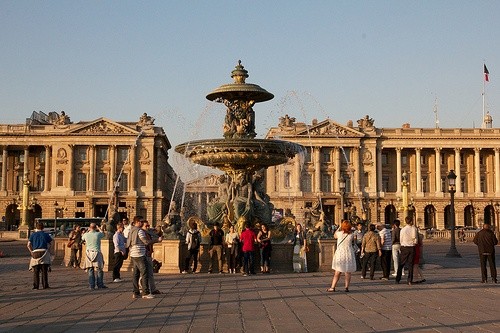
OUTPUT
[493,280,496,283]
[413,281,419,284]
[142,293,156,299]
[380,277,389,280]
[327,288,335,291]
[407,282,412,285]
[32,288,37,290]
[419,279,426,282]
[391,274,397,276]
[90,287,95,290]
[151,290,161,295]
[361,276,364,279]
[395,281,399,284]
[98,285,108,288]
[480,280,486,283]
[132,293,142,298]
[113,278,124,283]
[182,266,270,276]
[345,287,350,292]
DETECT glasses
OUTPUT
[144,225,149,227]
[138,220,143,223]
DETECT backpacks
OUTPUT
[189,231,200,250]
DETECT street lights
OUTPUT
[445,169,461,258]
[53,201,58,238]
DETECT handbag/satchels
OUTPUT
[151,258,162,273]
[67,239,75,248]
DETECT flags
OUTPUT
[485,65,490,82]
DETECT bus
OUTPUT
[33,217,106,237]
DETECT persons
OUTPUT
[26,216,498,300]
[214,172,268,234]
